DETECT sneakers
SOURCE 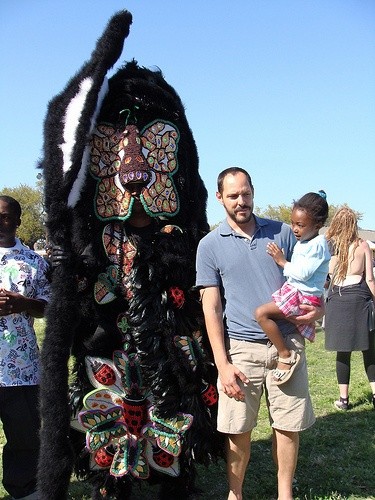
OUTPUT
[334,400,350,412]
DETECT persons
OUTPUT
[0,195,54,500]
[321,207,375,410]
[192,166,326,500]
[254,189,331,387]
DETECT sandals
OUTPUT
[268,351,300,385]
